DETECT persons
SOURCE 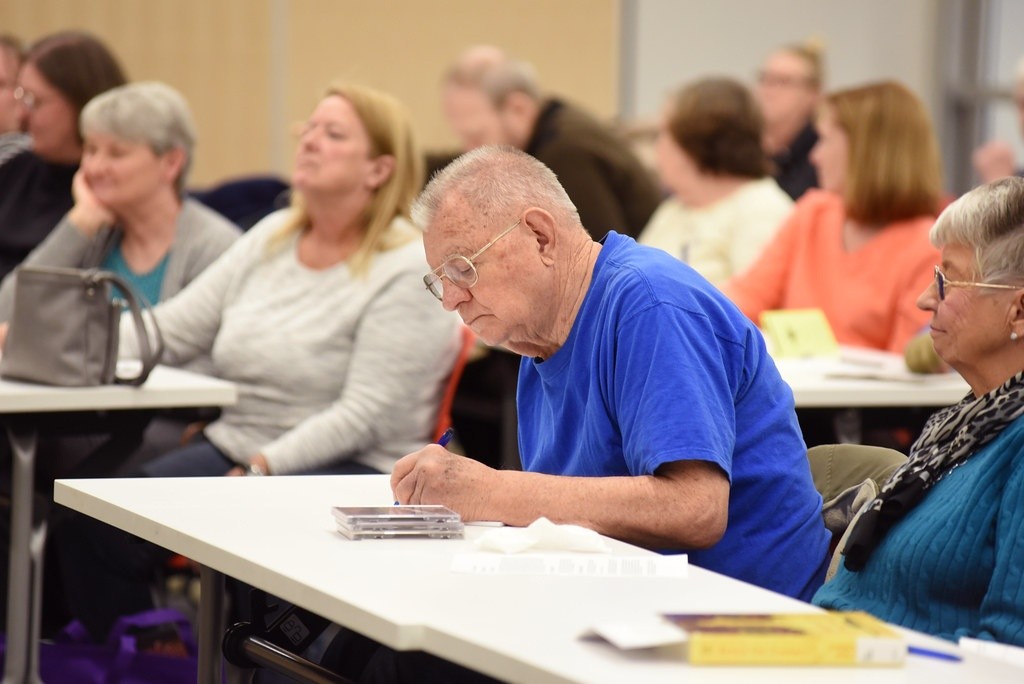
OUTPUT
[1,83,465,644]
[440,42,662,245]
[975,67,1024,185]
[810,174,1023,649]
[635,76,800,285]
[749,41,821,201]
[0,31,128,283]
[320,146,830,684]
[718,80,948,354]
[0,81,243,603]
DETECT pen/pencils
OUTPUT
[393,427,456,505]
[908,646,961,663]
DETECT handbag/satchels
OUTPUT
[0,267,164,386]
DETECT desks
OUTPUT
[770,348,972,452]
[0,354,237,684]
[51,474,965,684]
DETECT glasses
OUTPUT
[933,264,1024,300]
[422,223,523,301]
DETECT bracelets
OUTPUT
[240,460,262,477]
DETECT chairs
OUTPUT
[806,444,909,556]
